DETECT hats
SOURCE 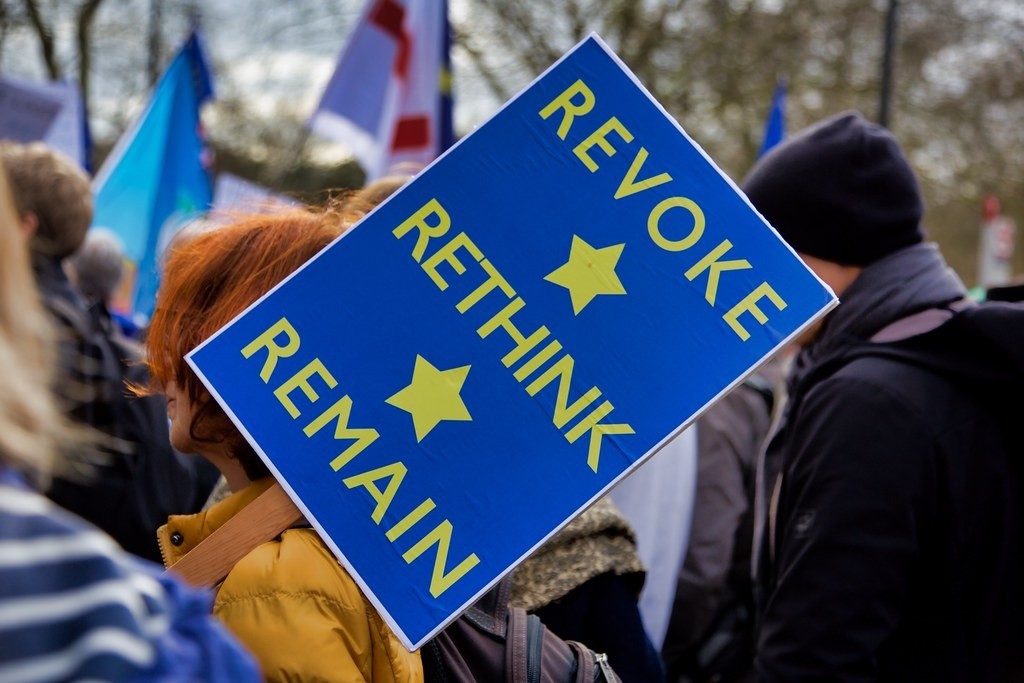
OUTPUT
[740,108,923,270]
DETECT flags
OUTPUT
[90,36,216,331]
[320,0,462,167]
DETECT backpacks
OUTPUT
[290,517,623,683]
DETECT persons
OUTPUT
[710,111,1024,683]
[0,134,775,683]
